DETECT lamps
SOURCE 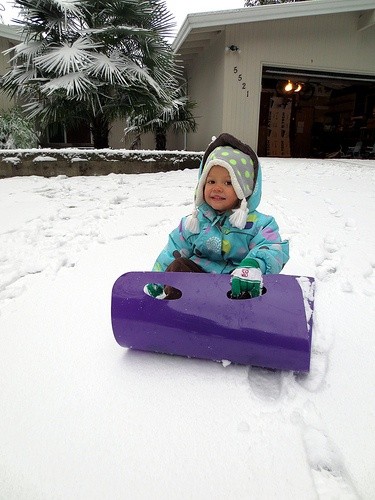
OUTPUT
[228,45,238,51]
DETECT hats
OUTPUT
[186,146,254,234]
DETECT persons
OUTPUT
[146,131,293,300]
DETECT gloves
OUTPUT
[147,284,164,297]
[230,258,261,297]
